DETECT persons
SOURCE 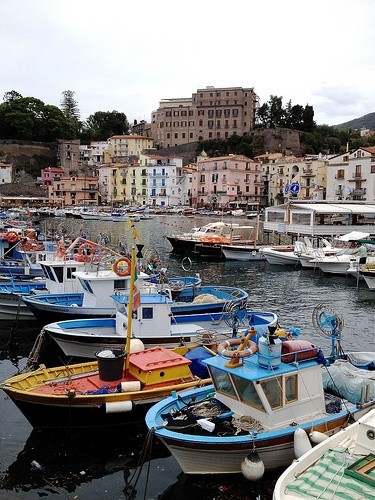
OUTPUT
[351,242,367,266]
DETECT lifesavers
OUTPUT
[78,242,95,255]
[217,338,257,357]
[56,241,65,258]
[113,257,131,276]
[28,222,32,228]
[6,231,18,242]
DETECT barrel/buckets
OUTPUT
[282,339,317,364]
[244,324,275,344]
[257,332,283,366]
[94,348,126,382]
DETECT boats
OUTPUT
[143,301,375,477]
[0,220,300,435]
[271,408,375,500]
[0,194,375,360]
